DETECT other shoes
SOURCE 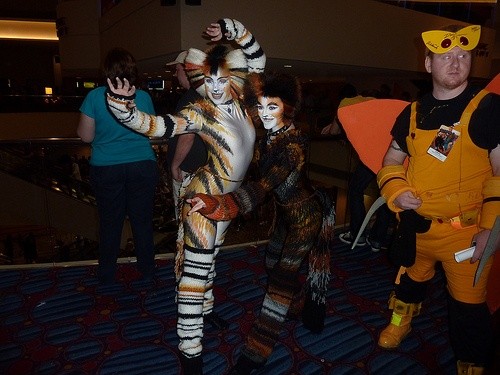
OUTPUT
[177,354,205,375]
[302,302,327,335]
[225,345,269,375]
[98,265,118,286]
[137,266,159,275]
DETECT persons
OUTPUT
[379,24,500,375]
[165,48,207,226]
[321,84,394,252]
[77,48,158,283]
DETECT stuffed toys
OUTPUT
[106,19,266,375]
[185,68,334,375]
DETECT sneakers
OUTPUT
[339,232,365,246]
[366,236,379,251]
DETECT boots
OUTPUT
[378,295,416,349]
[456,360,484,375]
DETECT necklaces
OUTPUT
[266,122,291,145]
[221,98,233,116]
